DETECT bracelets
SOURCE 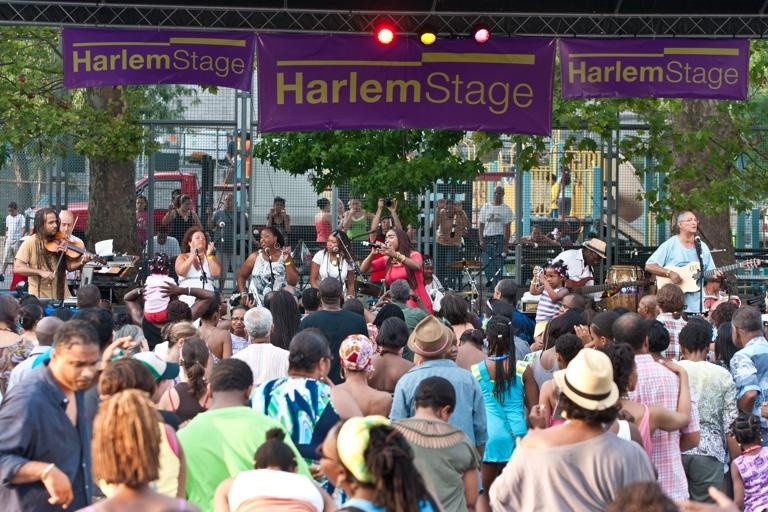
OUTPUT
[240,292,247,298]
[401,255,406,262]
[284,261,291,266]
[530,281,537,285]
[607,290,610,297]
[391,252,400,263]
[346,295,355,299]
[187,286,191,295]
[666,270,672,277]
[81,261,84,266]
[207,255,214,259]
[42,462,54,478]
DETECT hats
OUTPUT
[582,238,608,258]
[339,334,373,371]
[407,315,455,358]
[130,352,179,384]
[553,349,619,411]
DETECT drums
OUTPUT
[605,265,646,314]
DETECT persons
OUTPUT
[136,195,149,251]
[344,197,373,267]
[206,193,261,295]
[266,196,290,255]
[546,173,560,221]
[336,198,346,234]
[1,201,25,281]
[433,199,470,291]
[406,223,417,244]
[370,198,403,250]
[315,198,342,249]
[2,208,767,512]
[478,186,512,298]
[167,189,184,212]
[162,194,201,247]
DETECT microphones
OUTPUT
[332,246,340,253]
[694,235,705,255]
[485,267,501,287]
[265,246,272,260]
[219,221,225,242]
[361,240,384,248]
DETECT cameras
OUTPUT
[384,198,392,207]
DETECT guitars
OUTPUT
[563,276,654,299]
[656,258,761,295]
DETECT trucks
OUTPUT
[27,131,325,249]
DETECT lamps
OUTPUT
[471,23,491,45]
[417,25,436,46]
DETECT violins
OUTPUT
[43,231,107,266]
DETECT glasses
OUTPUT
[315,443,337,463]
[487,298,497,311]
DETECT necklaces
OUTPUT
[488,356,509,359]
[380,350,398,356]
[744,445,761,453]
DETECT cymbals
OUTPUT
[345,278,383,296]
[447,259,480,268]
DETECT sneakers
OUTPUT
[0,274,4,281]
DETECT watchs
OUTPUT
[459,211,463,215]
